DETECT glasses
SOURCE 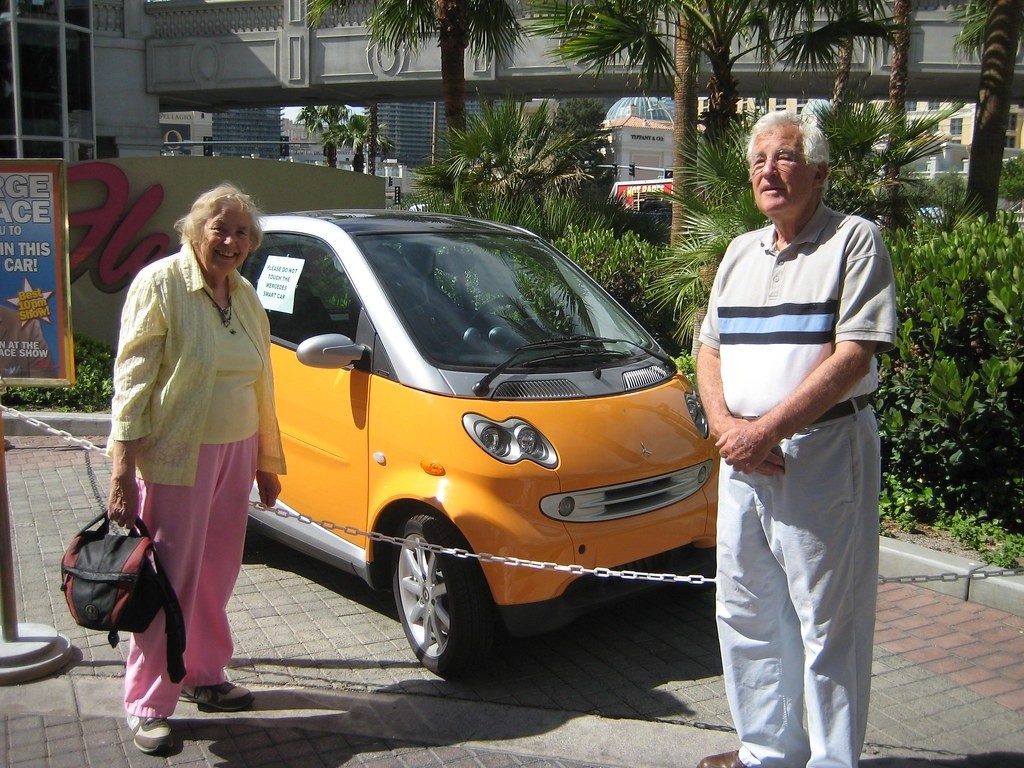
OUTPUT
[747,149,813,166]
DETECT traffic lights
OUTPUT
[611,161,621,178]
[628,163,637,178]
[394,186,401,206]
[663,169,673,178]
[388,176,394,187]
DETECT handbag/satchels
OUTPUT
[61,511,186,684]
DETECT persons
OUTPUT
[693,108,898,766]
[105,180,282,754]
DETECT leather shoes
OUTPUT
[696,750,748,768]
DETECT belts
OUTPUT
[732,393,869,424]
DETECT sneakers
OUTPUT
[178,681,254,710]
[127,713,174,752]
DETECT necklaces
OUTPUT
[202,287,237,335]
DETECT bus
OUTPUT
[605,180,706,213]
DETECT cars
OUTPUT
[235,206,725,678]
[914,206,949,232]
